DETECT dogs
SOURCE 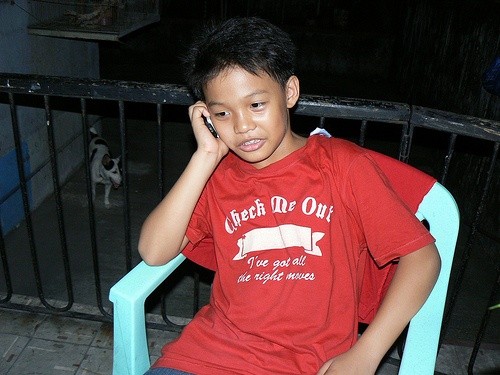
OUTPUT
[88,126,123,210]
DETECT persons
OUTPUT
[138,18,441,375]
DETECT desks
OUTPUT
[27,12,160,42]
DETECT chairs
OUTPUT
[111,149,460,375]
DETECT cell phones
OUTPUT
[202,110,219,138]
